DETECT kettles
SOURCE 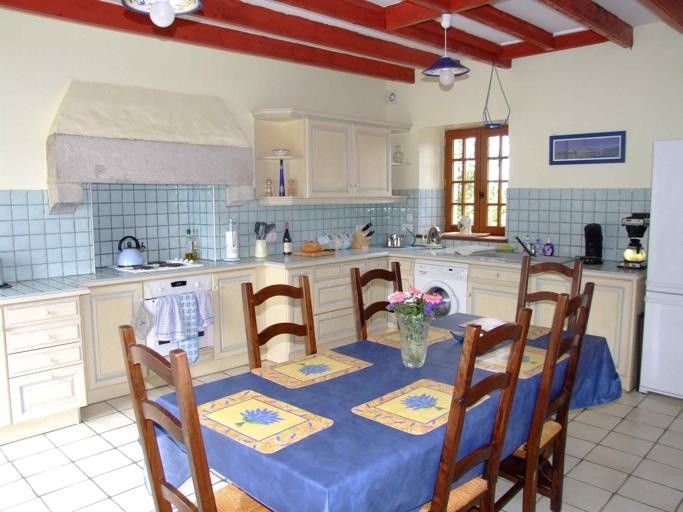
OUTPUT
[426,224,442,249]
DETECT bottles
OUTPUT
[278,160,285,196]
[287,178,295,196]
[281,221,292,255]
[263,179,272,197]
[392,143,404,164]
[184,229,196,261]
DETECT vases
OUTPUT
[394,312,433,370]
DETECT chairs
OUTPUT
[419,315,530,512]
[234,272,321,364]
[487,279,598,512]
[340,264,406,347]
[509,251,584,490]
[110,321,277,512]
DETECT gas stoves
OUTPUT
[108,257,202,274]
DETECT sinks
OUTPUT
[470,251,575,265]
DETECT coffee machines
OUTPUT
[616,211,650,269]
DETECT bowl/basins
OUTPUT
[271,149,289,157]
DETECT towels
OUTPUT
[149,295,187,342]
[459,311,555,343]
[351,378,492,437]
[252,348,373,388]
[195,286,214,330]
[469,341,573,380]
[175,292,200,366]
[185,384,336,455]
[359,324,462,351]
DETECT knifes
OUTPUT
[361,222,375,238]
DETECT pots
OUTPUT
[385,233,404,247]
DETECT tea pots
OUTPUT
[115,234,145,267]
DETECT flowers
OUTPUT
[386,280,452,319]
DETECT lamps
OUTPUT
[422,12,468,88]
[115,0,203,32]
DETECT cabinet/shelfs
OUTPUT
[250,105,412,206]
[289,254,390,365]
[465,263,530,338]
[210,271,268,371]
[81,283,149,405]
[385,254,415,329]
[529,264,646,392]
[0,293,86,442]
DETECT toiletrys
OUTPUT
[543,237,554,256]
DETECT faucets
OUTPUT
[512,235,535,256]
[404,225,416,246]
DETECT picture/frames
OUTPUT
[549,130,627,165]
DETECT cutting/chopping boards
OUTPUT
[294,251,335,257]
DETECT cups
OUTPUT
[254,240,268,258]
[226,232,240,259]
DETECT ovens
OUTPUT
[144,293,213,356]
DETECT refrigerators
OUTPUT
[637,140,683,400]
